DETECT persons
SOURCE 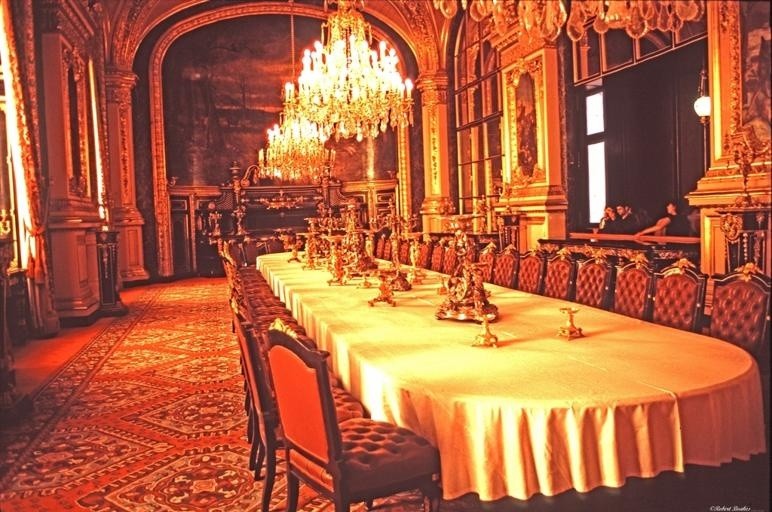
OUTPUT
[591,196,701,251]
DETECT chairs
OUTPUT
[216,228,771,511]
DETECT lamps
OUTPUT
[430,0,708,45]
[283,1,416,141]
[693,68,710,125]
[256,1,337,185]
